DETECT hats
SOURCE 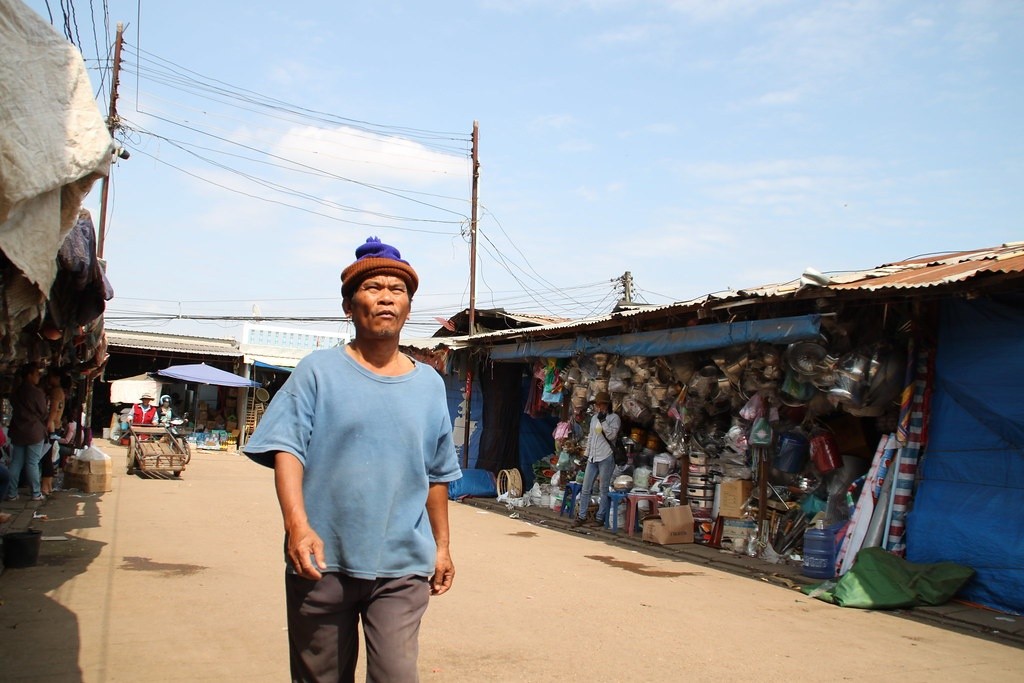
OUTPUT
[140,394,154,400]
[341,236,419,299]
[596,392,610,402]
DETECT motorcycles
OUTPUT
[159,411,191,464]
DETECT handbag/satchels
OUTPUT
[612,436,628,466]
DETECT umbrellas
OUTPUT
[147,361,263,435]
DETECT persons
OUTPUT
[0,405,12,501]
[571,409,593,436]
[126,392,159,470]
[242,236,462,683]
[6,362,48,501]
[570,391,621,528]
[837,411,899,462]
[157,394,188,425]
[40,367,73,497]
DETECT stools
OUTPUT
[605,492,629,533]
[624,494,658,537]
[561,484,582,518]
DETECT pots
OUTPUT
[534,340,900,455]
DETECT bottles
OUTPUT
[804,520,837,579]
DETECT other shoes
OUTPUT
[591,521,604,527]
[32,494,44,500]
[9,495,19,500]
[572,518,583,526]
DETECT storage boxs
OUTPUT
[720,519,758,554]
[719,481,754,518]
[641,504,694,546]
[65,457,112,494]
[195,389,238,433]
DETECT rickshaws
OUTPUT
[127,421,186,477]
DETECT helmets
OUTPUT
[161,395,171,405]
